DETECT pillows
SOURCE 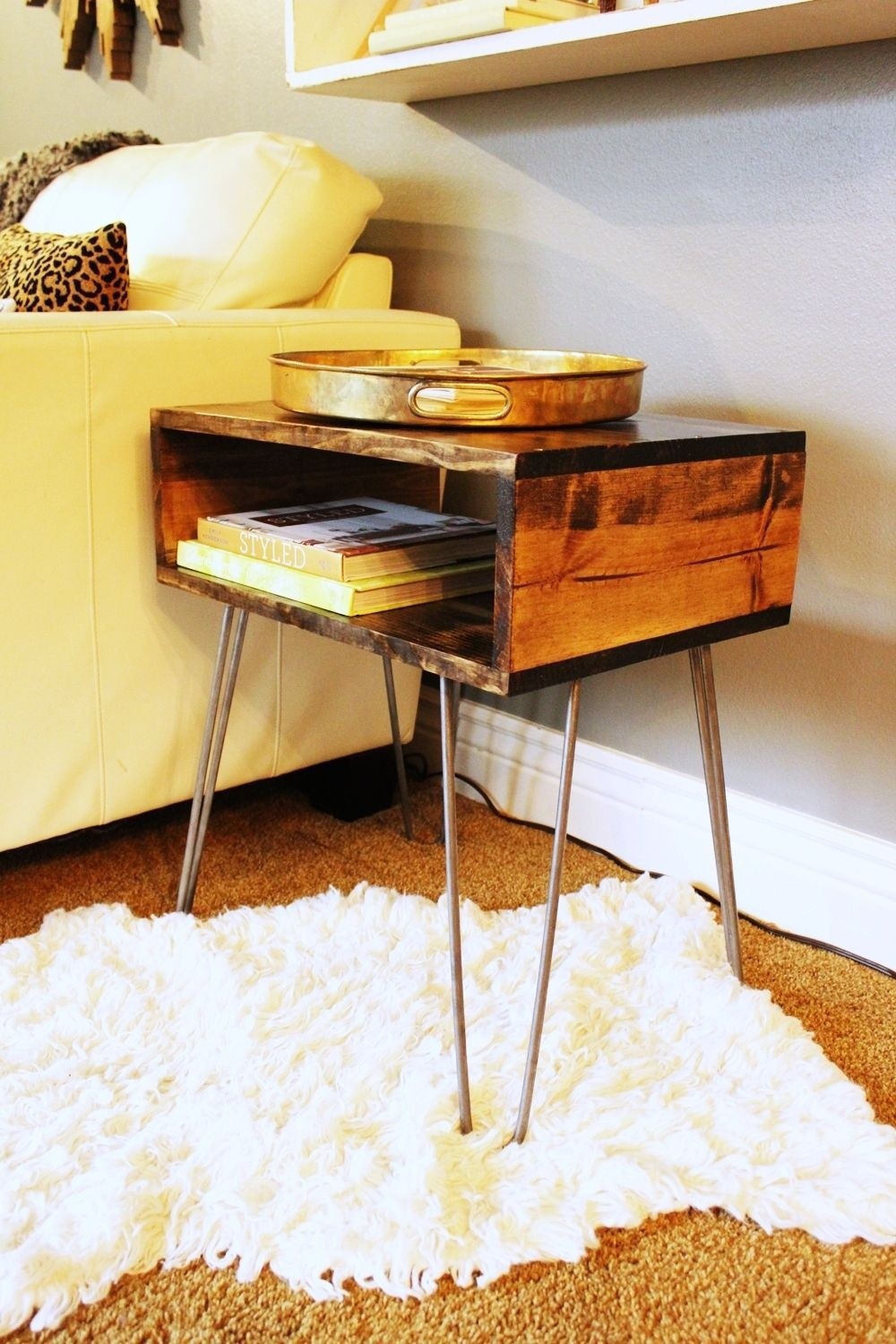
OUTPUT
[0,220,130,311]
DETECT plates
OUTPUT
[270,352,647,428]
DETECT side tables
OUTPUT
[152,397,802,1156]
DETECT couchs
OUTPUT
[0,126,462,885]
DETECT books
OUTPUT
[175,493,496,617]
[367,1,601,57]
